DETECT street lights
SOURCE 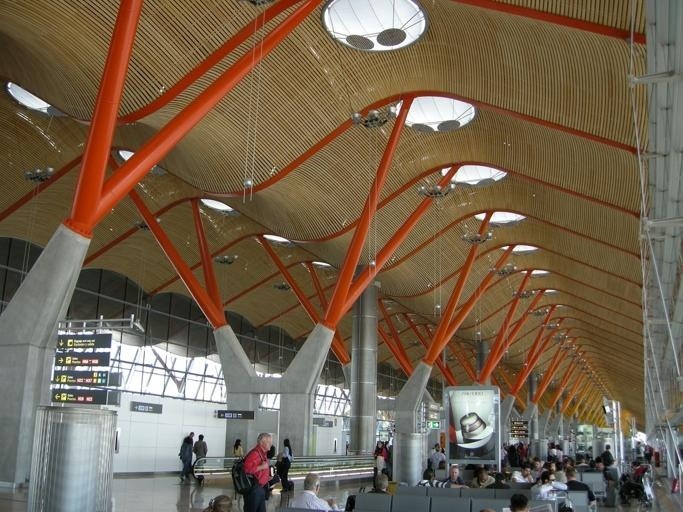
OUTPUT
[600,398,620,459]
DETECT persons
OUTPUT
[287,473,342,511]
[240,432,272,511]
[201,494,232,511]
[267,445,276,477]
[276,438,294,492]
[192,434,207,472]
[366,439,656,511]
[232,438,245,464]
[187,432,194,473]
[177,436,192,482]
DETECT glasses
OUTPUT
[548,479,556,482]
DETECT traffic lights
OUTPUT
[432,421,440,429]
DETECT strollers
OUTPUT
[618,473,652,508]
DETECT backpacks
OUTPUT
[231,448,263,502]
